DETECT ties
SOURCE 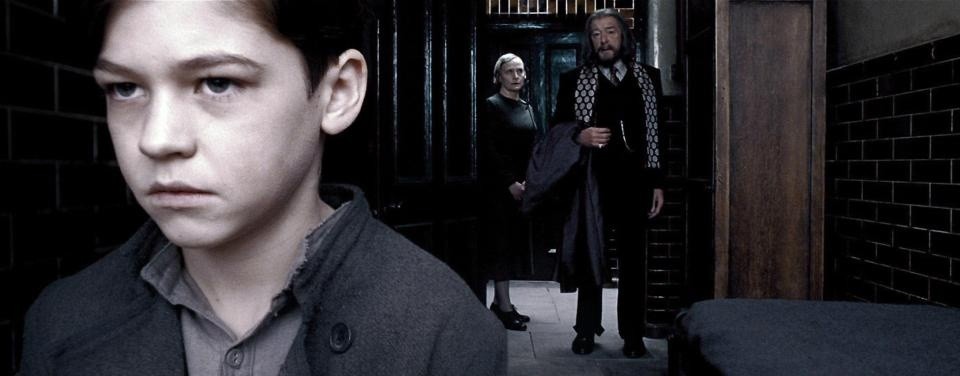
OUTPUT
[611,66,620,86]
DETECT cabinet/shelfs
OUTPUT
[676,0,829,300]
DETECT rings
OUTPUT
[598,144,603,149]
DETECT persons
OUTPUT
[18,0,509,376]
[476,53,538,332]
[549,8,670,360]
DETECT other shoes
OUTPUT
[573,333,595,355]
[490,302,529,322]
[496,307,527,331]
[622,337,646,358]
